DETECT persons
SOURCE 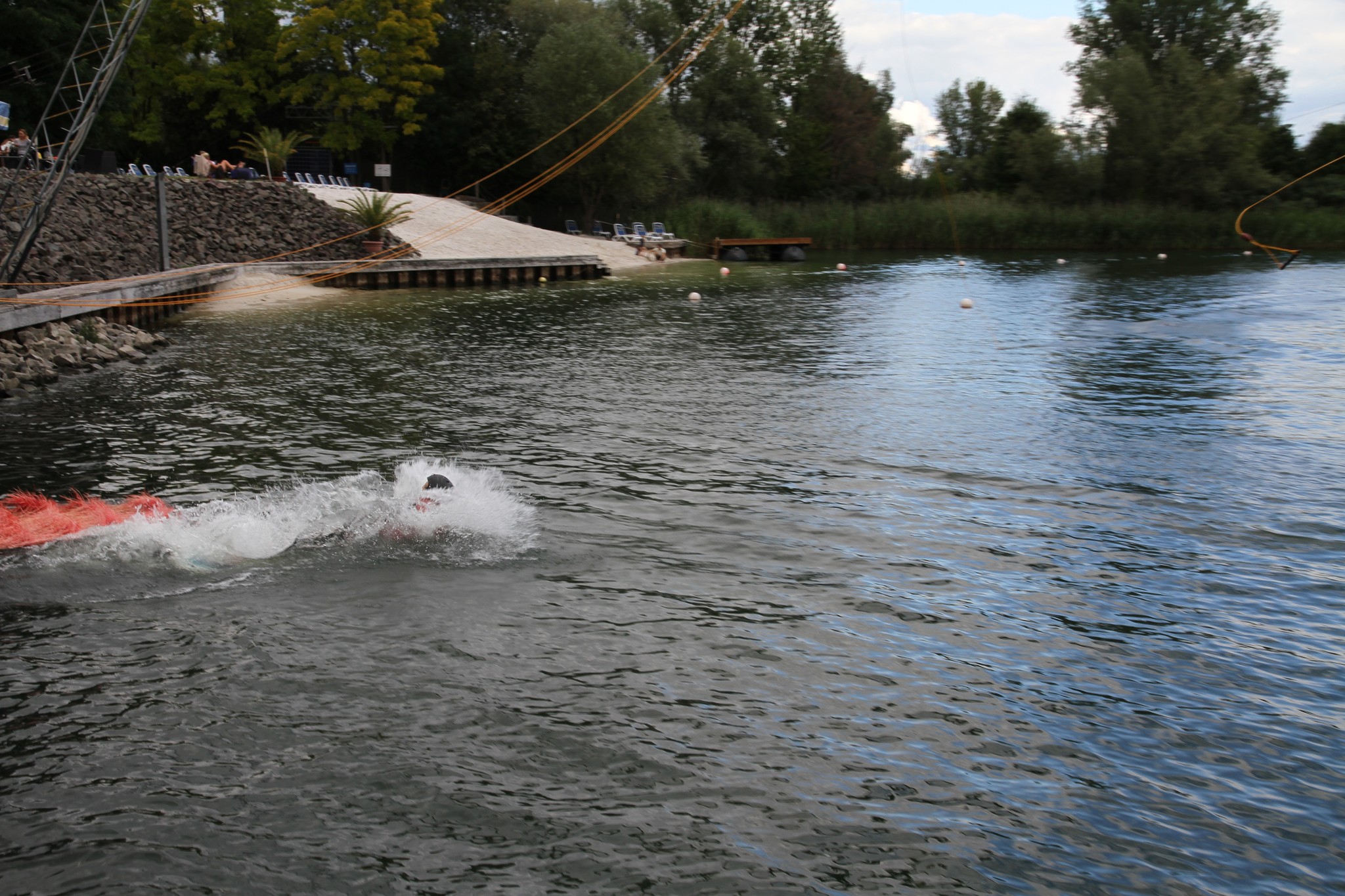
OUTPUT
[0,129,61,170]
[634,234,666,262]
[378,475,455,543]
[200,150,244,175]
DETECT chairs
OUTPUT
[566,220,581,234]
[282,171,351,187]
[593,220,674,241]
[116,164,188,176]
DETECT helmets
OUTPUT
[425,473,454,491]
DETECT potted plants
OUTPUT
[337,187,416,253]
